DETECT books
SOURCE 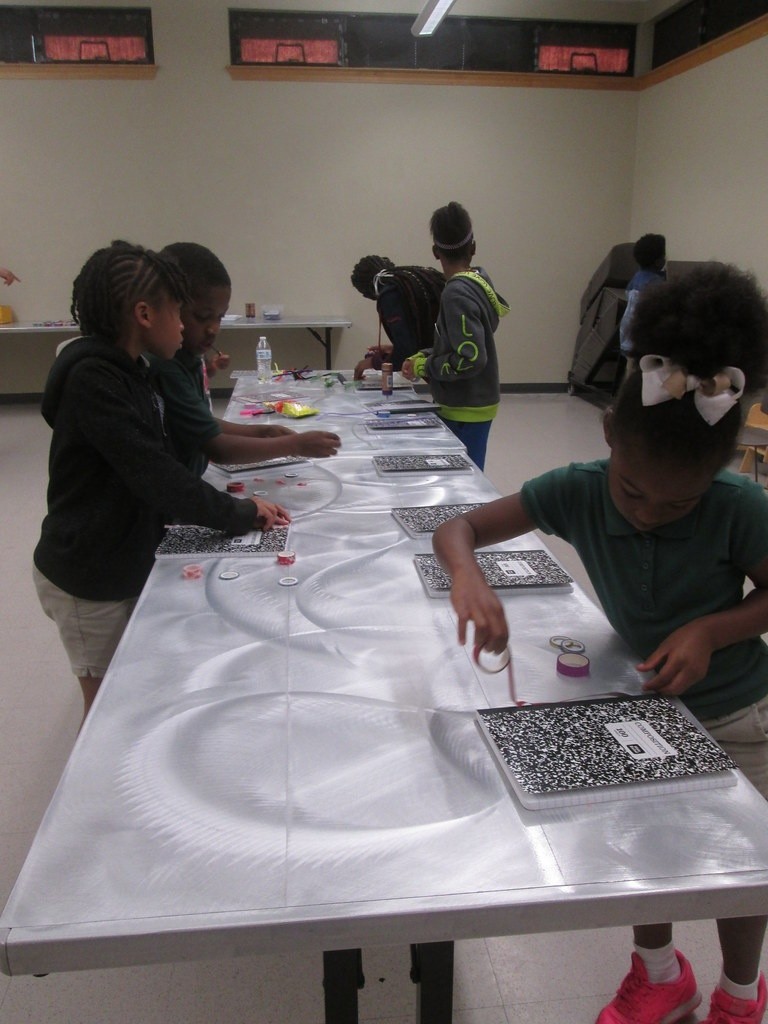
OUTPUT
[476,694,739,811]
[155,524,292,557]
[372,454,475,477]
[391,503,490,539]
[231,389,311,404]
[356,383,443,430]
[414,549,575,599]
[207,455,313,479]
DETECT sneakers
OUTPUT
[700,969,768,1024]
[594,948,702,1024]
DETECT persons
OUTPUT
[34,237,290,744]
[432,264,768,1024]
[141,240,341,477]
[401,200,511,474]
[0,265,22,286]
[601,233,668,420]
[350,254,445,384]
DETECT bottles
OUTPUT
[255,336,273,385]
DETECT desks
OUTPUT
[1,320,354,371]
[0,353,768,1024]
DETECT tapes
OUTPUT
[377,410,391,417]
[277,550,296,565]
[227,482,244,493]
[284,473,298,478]
[279,577,298,586]
[471,640,513,676]
[555,653,591,678]
[253,490,268,497]
[183,564,202,579]
[548,634,585,653]
[219,571,239,580]
[33,320,76,327]
